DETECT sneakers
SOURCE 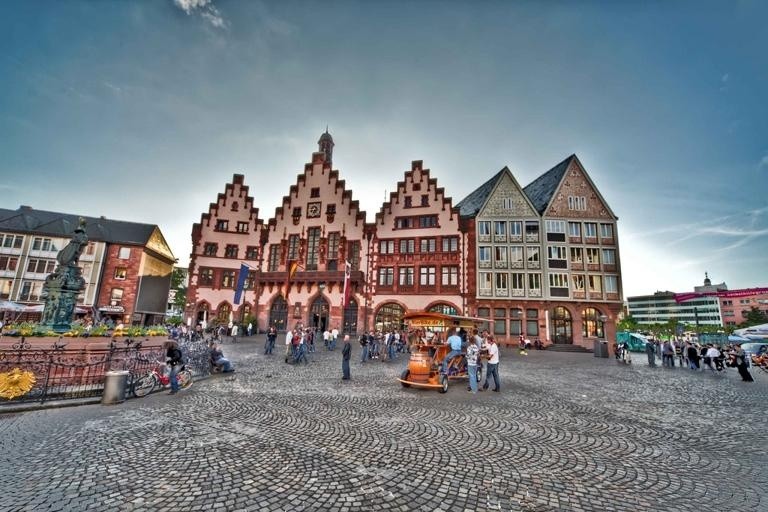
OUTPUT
[519,350,529,356]
[340,374,351,381]
[613,356,756,382]
[262,347,411,364]
[221,367,236,375]
[464,384,503,395]
[167,388,179,396]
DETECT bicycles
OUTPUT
[131,351,194,398]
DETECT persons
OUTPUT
[197,319,503,396]
[519,331,525,355]
[161,340,186,396]
[612,331,767,383]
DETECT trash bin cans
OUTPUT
[594,337,609,359]
[101,370,129,406]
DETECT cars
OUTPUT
[614,323,768,367]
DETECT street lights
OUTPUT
[237,277,250,338]
[314,283,325,344]
[348,300,355,334]
[599,313,609,342]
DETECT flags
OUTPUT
[233,262,249,307]
[341,260,353,309]
[284,260,299,301]
